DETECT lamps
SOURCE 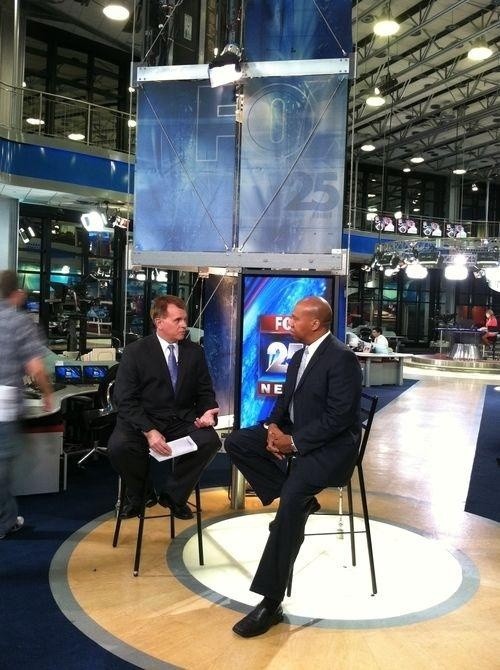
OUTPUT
[206,42,243,88]
[362,253,500,297]
[357,0,500,188]
[98,0,135,25]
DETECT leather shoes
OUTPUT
[158,490,194,520]
[120,488,157,519]
[233,604,285,637]
[268,495,321,534]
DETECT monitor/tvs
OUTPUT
[83,366,108,382]
[55,365,81,382]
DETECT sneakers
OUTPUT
[1,515,25,541]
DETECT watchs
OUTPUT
[291,436,298,454]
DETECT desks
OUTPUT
[354,351,413,387]
[436,327,500,363]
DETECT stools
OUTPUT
[106,454,204,575]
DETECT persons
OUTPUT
[371,327,388,353]
[0,270,52,539]
[108,295,222,519]
[482,309,497,350]
[224,296,363,638]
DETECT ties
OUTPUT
[289,346,307,423]
[164,343,182,392]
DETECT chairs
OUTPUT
[279,388,381,601]
[62,361,128,466]
